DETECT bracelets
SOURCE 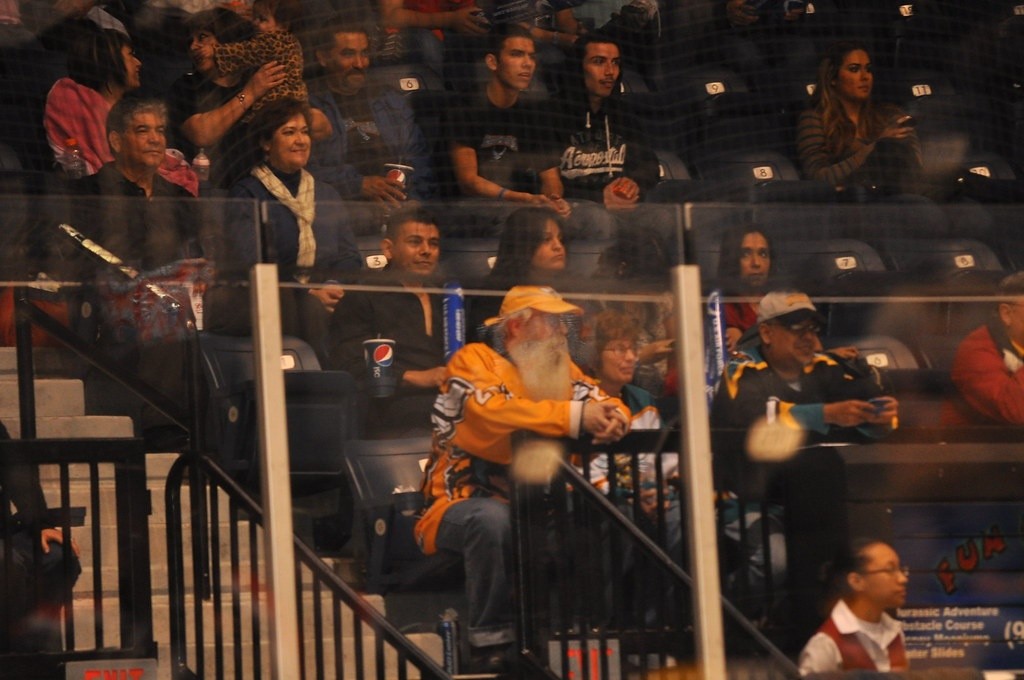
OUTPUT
[498,186,507,198]
[551,30,560,44]
[237,91,248,112]
[527,24,535,32]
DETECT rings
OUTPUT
[71,539,75,543]
[622,209,624,214]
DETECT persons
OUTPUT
[414,284,632,673]
[711,285,899,594]
[193,0,309,171]
[305,16,439,214]
[538,31,675,250]
[581,236,678,392]
[476,207,602,324]
[645,293,680,403]
[698,0,819,91]
[795,34,923,189]
[168,6,332,189]
[704,221,778,354]
[486,0,588,44]
[329,208,491,513]
[444,22,619,239]
[0,420,83,653]
[951,271,1024,429]
[43,27,198,199]
[551,306,682,670]
[33,85,238,453]
[0,0,138,193]
[379,0,497,63]
[796,532,910,677]
[203,92,362,372]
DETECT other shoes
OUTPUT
[474,652,507,672]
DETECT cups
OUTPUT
[381,163,415,217]
[363,340,396,397]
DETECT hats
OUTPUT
[482,285,584,328]
[757,290,825,325]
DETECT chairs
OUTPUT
[0,0,1024,586]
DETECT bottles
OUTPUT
[64,139,88,181]
[191,148,211,181]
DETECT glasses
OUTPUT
[861,565,908,578]
[768,321,821,334]
[604,345,639,358]
